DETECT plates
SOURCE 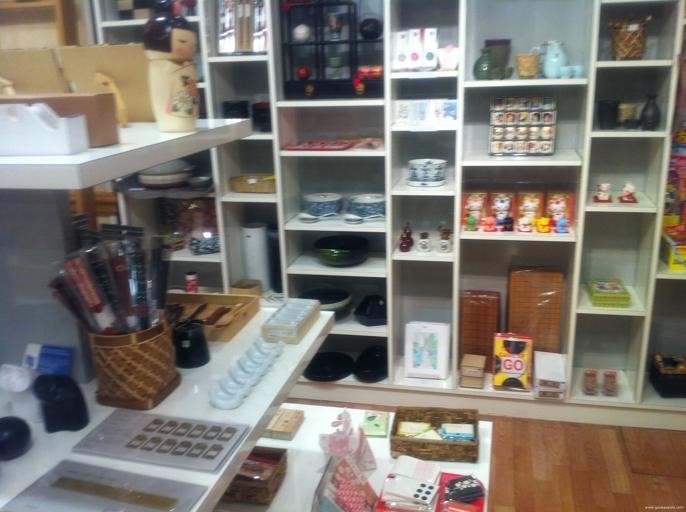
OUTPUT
[405,179,446,186]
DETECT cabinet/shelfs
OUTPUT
[565,3,684,424]
[93,1,226,298]
[451,0,598,423]
[637,36,685,426]
[386,1,463,407]
[201,2,284,302]
[272,1,390,405]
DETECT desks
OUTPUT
[0,115,336,511]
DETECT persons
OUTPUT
[518,217,533,231]
[546,197,567,219]
[465,217,476,231]
[145,1,201,132]
[554,216,569,233]
[503,218,514,231]
[483,216,497,231]
[465,195,484,225]
[184,271,199,294]
[536,218,551,232]
[519,196,540,218]
[492,197,512,225]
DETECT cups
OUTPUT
[573,66,584,77]
[504,66,512,76]
[561,67,574,78]
[515,54,540,78]
[625,119,639,129]
[617,104,636,126]
[598,100,619,130]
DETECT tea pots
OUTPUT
[529,41,569,78]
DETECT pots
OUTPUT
[138,161,195,188]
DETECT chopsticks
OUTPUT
[64,256,115,337]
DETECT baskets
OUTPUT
[222,446,287,505]
[230,174,276,193]
[649,360,686,398]
[389,406,479,462]
[608,21,646,60]
[89,308,182,409]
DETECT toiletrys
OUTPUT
[442,229,453,244]
[439,233,450,252]
[416,232,430,251]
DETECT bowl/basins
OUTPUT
[189,177,210,188]
[301,287,353,320]
[346,193,386,218]
[408,159,447,181]
[314,235,370,266]
[302,193,344,215]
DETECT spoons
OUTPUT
[344,214,384,223]
[300,212,337,223]
